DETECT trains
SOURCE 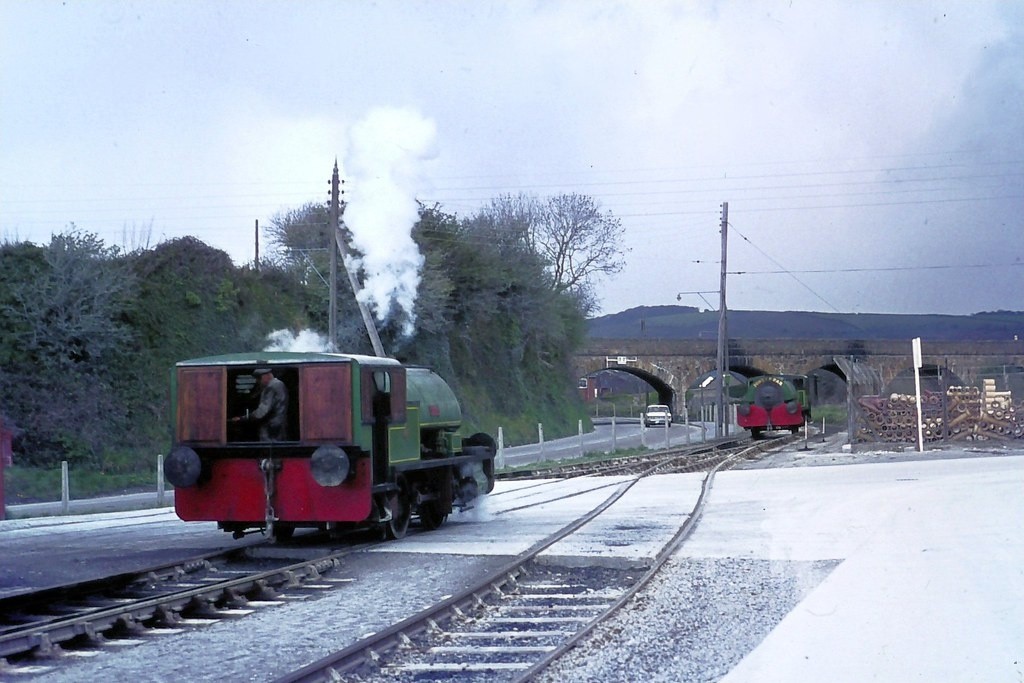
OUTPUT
[161,352,496,540]
[736,373,810,438]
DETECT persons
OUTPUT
[231,369,288,442]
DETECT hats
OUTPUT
[254,368,273,375]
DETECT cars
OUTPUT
[644,404,673,427]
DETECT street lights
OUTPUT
[678,290,731,436]
